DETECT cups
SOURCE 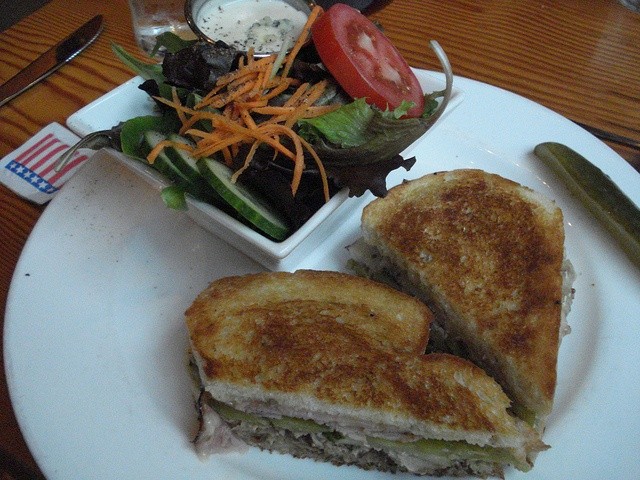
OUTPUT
[129,1,186,58]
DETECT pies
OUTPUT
[344,169,565,441]
[184,269,551,480]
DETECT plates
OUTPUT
[65,69,466,260]
[4,67,639,480]
[184,0,312,58]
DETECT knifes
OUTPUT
[535,142,639,267]
[0,15,104,107]
